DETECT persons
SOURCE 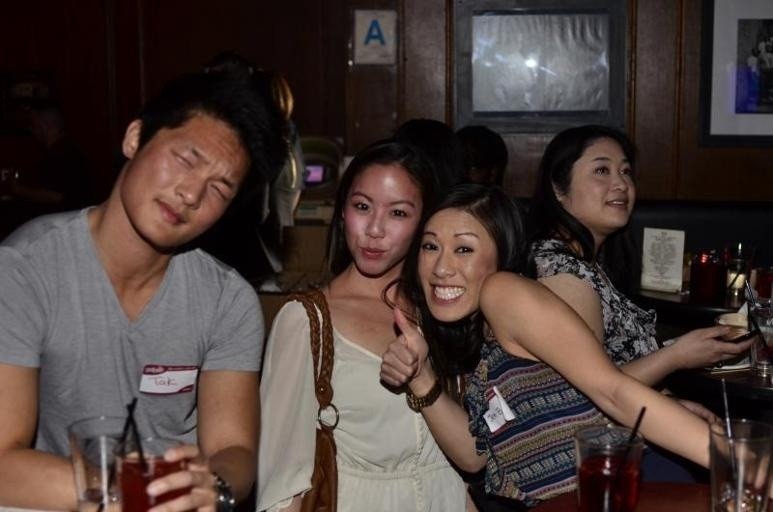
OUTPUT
[1,62,268,512]
[376,184,773,511]
[255,141,479,512]
[394,120,509,195]
[217,70,309,284]
[522,126,755,425]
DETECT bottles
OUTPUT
[725,227,748,309]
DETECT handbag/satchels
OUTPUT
[286,286,339,512]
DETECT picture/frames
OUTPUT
[696,0,773,149]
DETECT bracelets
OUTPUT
[408,375,442,408]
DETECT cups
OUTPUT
[65,415,129,512]
[746,300,772,376]
[714,313,747,343]
[570,426,643,512]
[113,437,189,512]
[707,419,772,512]
